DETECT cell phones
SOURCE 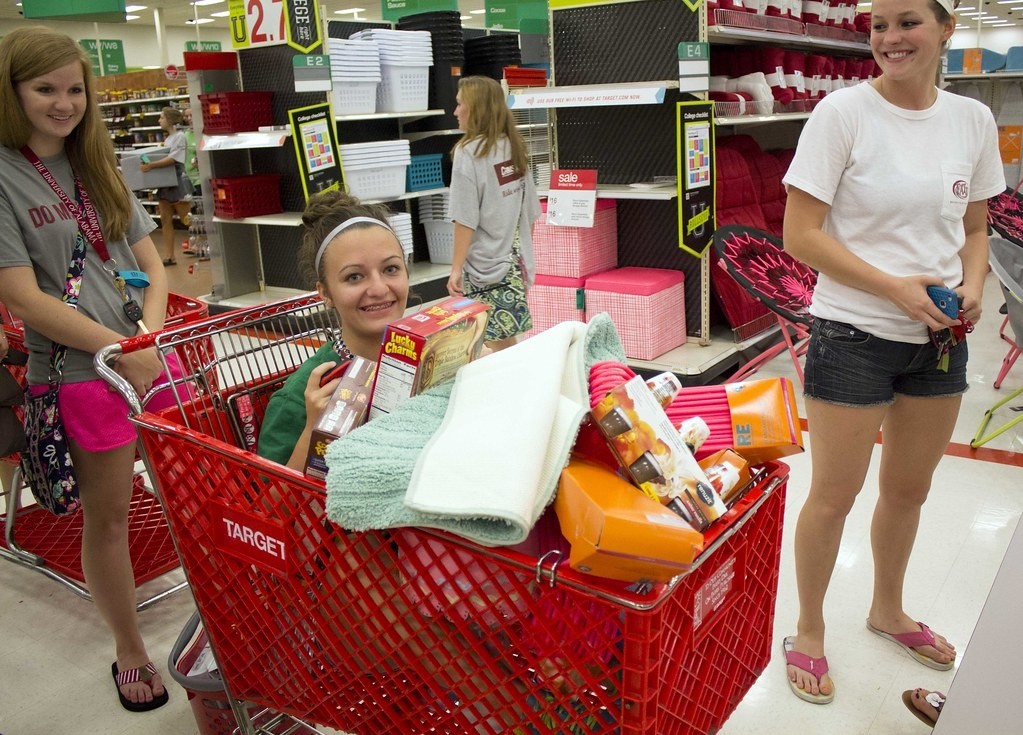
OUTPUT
[927,285,959,321]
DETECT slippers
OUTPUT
[902,688,947,728]
[784,636,835,704]
[112,659,168,713]
[867,616,955,671]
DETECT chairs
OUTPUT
[710,192,1022,448]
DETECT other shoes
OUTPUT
[183,250,205,257]
[163,258,177,266]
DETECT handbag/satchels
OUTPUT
[24,387,82,517]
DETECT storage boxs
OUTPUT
[207,172,282,219]
[322,9,522,272]
[514,270,615,339]
[579,264,689,359]
[367,294,489,422]
[529,197,620,278]
[197,86,274,132]
[552,454,704,580]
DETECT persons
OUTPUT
[446,75,541,354]
[139,106,196,267]
[256,191,620,735]
[782,0,1007,704]
[183,109,201,257]
[902,688,946,728]
[0,26,201,714]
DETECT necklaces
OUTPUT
[334,329,356,361]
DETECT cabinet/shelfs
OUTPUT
[492,0,880,387]
[182,19,537,318]
[93,89,208,230]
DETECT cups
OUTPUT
[703,461,740,500]
[666,496,693,524]
[629,450,664,484]
[646,371,683,411]
[600,405,632,438]
[674,416,711,455]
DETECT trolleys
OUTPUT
[0,290,220,615]
[89,288,792,734]
